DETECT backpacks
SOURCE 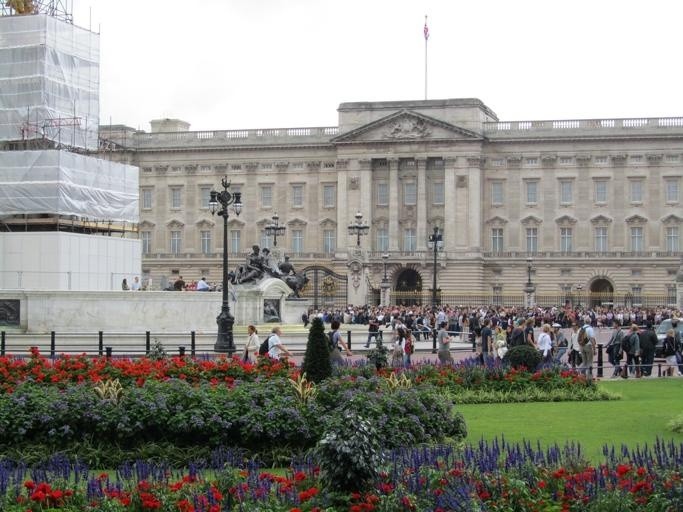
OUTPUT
[622,333,635,351]
[577,325,590,346]
[402,335,411,354]
[258,334,276,355]
[325,331,338,352]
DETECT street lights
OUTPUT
[347,211,392,282]
[208,173,243,351]
[576,280,582,306]
[427,225,443,304]
[495,283,500,308]
[525,259,535,282]
[264,210,286,248]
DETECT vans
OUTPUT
[654,318,683,353]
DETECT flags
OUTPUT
[424,24,429,39]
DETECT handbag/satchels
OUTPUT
[606,345,614,354]
[476,351,484,364]
[676,351,682,364]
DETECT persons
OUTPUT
[399,282,409,291]
[122,277,142,290]
[303,305,683,380]
[245,326,293,363]
[217,284,223,291]
[174,276,188,291]
[416,282,421,291]
[232,244,297,283]
[197,278,210,290]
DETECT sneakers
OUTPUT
[611,369,651,378]
[364,345,369,348]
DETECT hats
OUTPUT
[552,323,562,328]
